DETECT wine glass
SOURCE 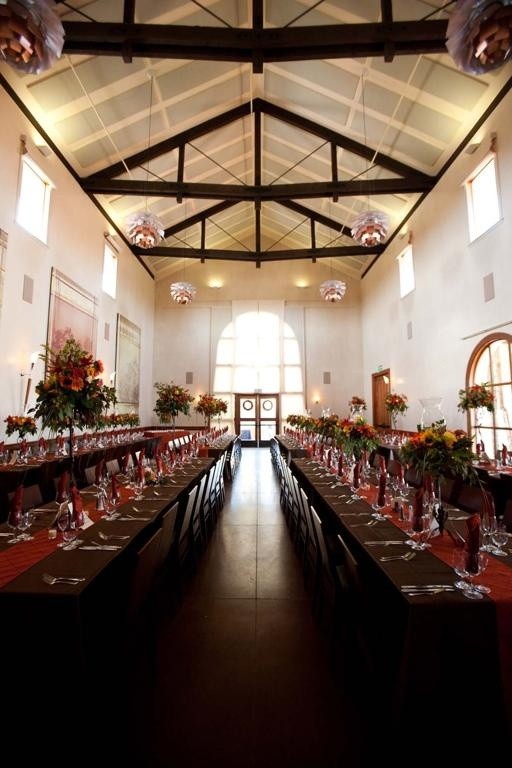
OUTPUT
[0,425,231,552]
[283,428,511,602]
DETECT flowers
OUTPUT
[286,382,496,498]
[4,335,227,438]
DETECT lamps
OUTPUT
[170,199,197,305]
[0,0,65,75]
[445,0,512,76]
[126,69,165,250]
[319,202,347,303]
[350,67,389,247]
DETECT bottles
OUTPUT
[418,397,447,433]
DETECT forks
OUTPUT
[41,571,86,587]
